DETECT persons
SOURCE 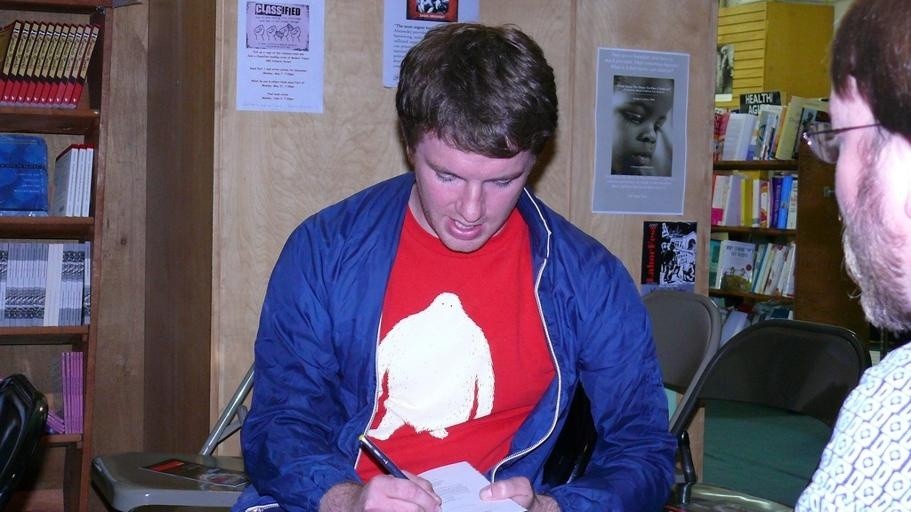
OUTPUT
[230,20,681,512]
[611,75,674,175]
[793,1,911,512]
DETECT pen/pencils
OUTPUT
[358,434,410,480]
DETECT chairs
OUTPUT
[667,318,873,511]
[543,290,722,485]
[91,363,255,512]
[0,374,48,512]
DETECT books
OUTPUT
[47,350,89,436]
[138,458,250,487]
[0,19,101,105]
[0,132,94,327]
[708,89,830,349]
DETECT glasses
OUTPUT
[802,122,882,164]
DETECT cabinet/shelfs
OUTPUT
[708,109,865,327]
[0,0,116,512]
